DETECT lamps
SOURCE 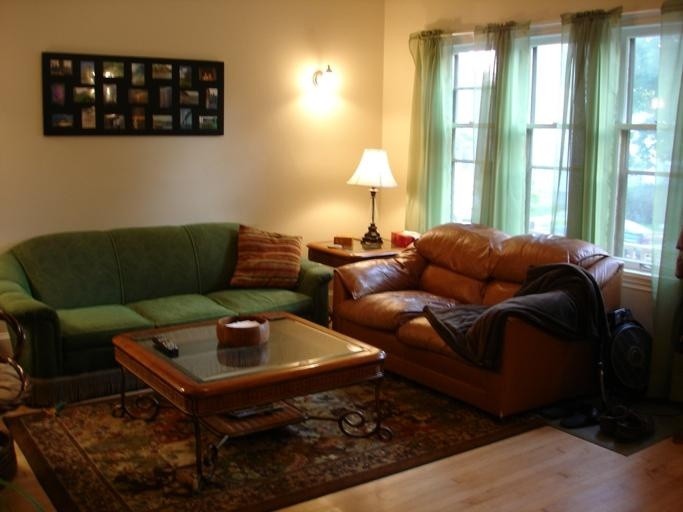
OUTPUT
[298,64,342,122]
[347,148,397,245]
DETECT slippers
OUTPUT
[542,403,602,428]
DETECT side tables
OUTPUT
[307,235,400,268]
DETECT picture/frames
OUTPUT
[41,50,225,137]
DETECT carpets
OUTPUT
[546,403,682,456]
[2,379,551,512]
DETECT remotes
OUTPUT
[152,334,179,357]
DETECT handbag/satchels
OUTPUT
[600,404,655,442]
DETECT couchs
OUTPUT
[0,222,332,408]
[332,223,624,417]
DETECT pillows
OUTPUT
[233,224,306,290]
